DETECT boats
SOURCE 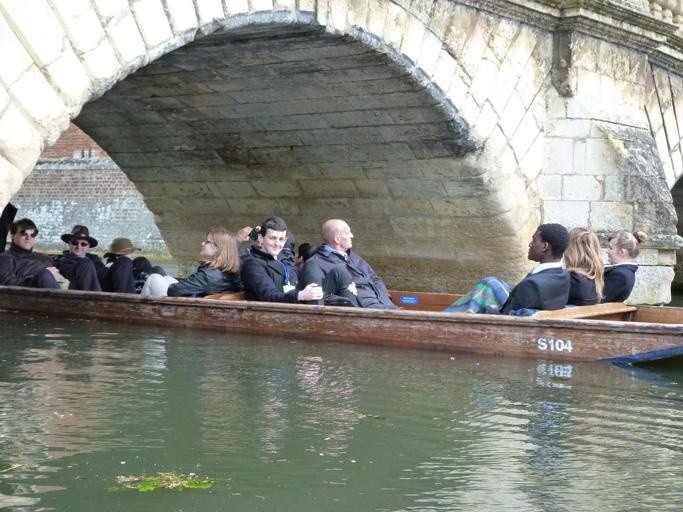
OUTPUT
[0,276,683,368]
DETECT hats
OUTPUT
[61,225,98,248]
[104,238,141,258]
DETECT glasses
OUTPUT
[205,240,216,245]
[20,231,35,238]
[71,240,88,247]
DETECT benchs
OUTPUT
[219,290,245,301]
[202,290,230,299]
[532,301,637,321]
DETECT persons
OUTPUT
[563,226,605,307]
[1,215,396,308]
[601,230,647,303]
[443,222,571,315]
